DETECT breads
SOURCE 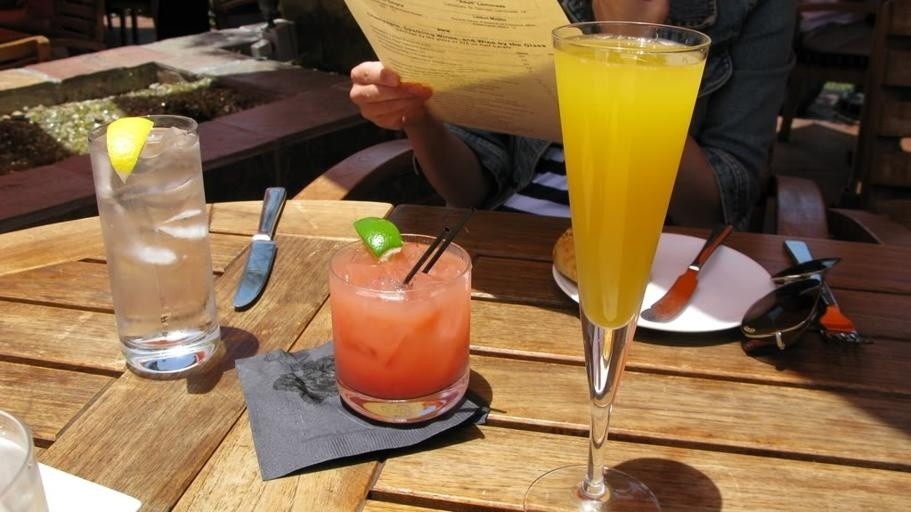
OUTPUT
[552,228,577,283]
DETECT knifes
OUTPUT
[650,223,737,318]
[233,187,287,308]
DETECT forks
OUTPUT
[784,240,862,343]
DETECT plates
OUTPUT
[548,227,779,335]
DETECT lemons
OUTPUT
[106,117,153,184]
[353,217,401,260]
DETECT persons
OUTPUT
[348,0,800,232]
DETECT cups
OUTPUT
[329,231,472,424]
[89,113,223,377]
[0,407,51,511]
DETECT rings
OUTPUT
[400,114,407,124]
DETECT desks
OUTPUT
[2,186,911,512]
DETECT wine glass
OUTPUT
[519,20,712,511]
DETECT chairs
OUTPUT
[289,128,836,241]
